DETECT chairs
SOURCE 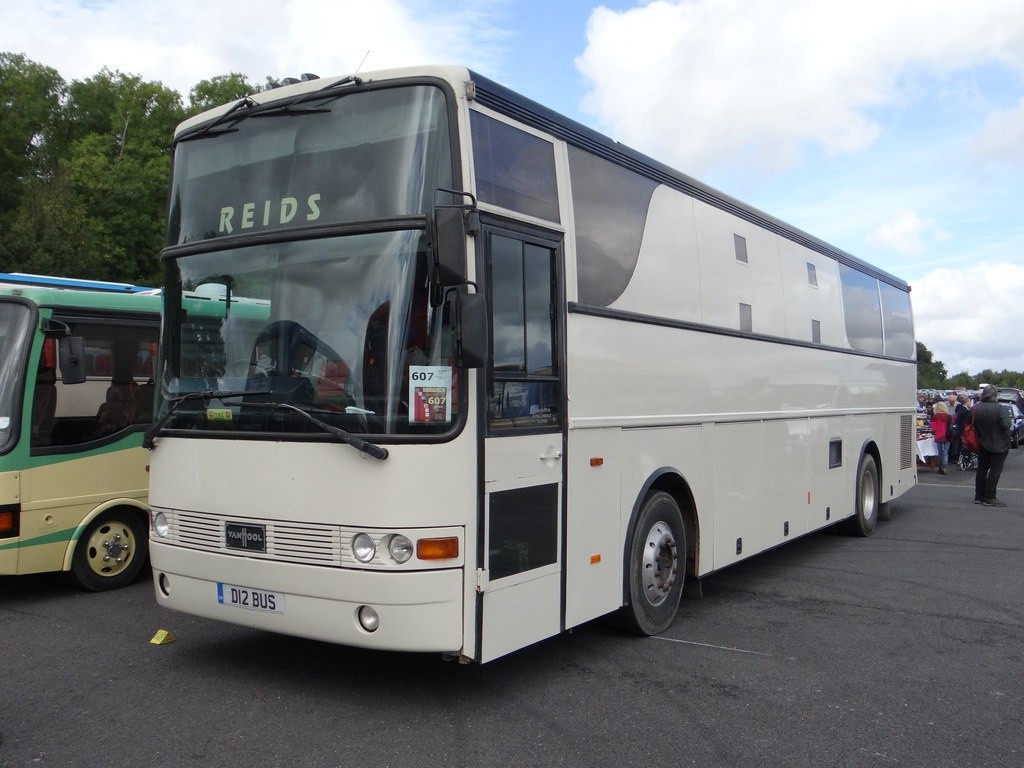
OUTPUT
[82,376,155,436]
[31,368,57,446]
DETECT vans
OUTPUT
[997,388,1022,411]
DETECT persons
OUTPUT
[973,385,1012,507]
[918,391,972,474]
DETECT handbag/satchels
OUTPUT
[948,420,959,436]
[960,404,979,452]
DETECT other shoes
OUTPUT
[939,466,946,474]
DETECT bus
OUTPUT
[55,337,158,416]
[142,65,920,668]
[0,273,271,592]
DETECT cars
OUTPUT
[998,402,1024,448]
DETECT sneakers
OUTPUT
[974,498,983,503]
[983,498,1006,507]
